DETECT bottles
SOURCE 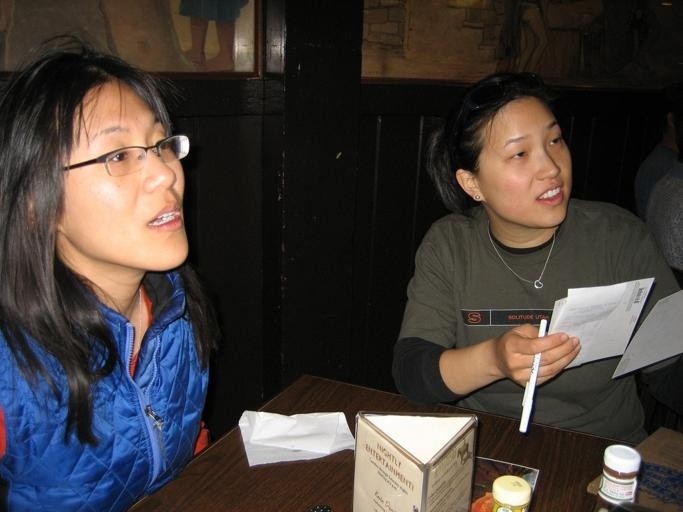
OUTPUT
[492,473,533,512]
[593,443,642,512]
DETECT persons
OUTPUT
[389,69,683,450]
[0,30,223,512]
[632,81,683,224]
[646,105,683,290]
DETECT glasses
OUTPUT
[452,72,544,124]
[64,133,190,178]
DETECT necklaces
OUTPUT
[138,288,143,349]
[485,220,556,288]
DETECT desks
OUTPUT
[124,374,682,512]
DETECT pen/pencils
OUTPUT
[518,319,547,433]
